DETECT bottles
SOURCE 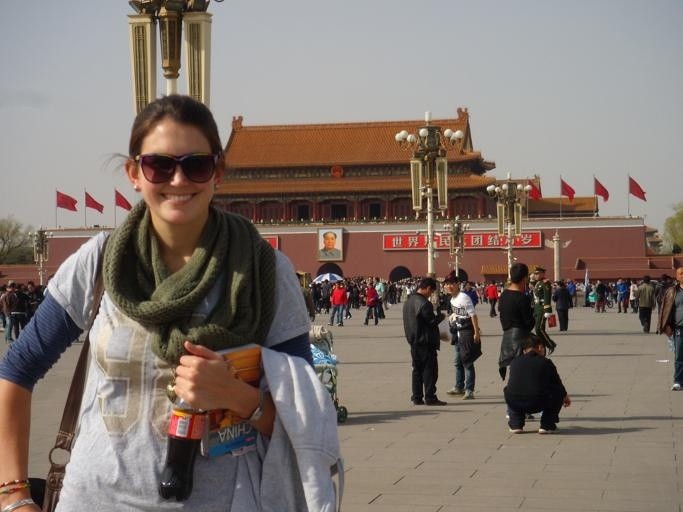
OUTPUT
[157,397,208,502]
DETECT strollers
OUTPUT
[309,326,346,424]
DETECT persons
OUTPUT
[319,231,341,260]
[656,266,682,391]
[477,280,506,317]
[549,274,679,332]
[402,277,448,406]
[297,272,424,325]
[0,95,345,511]
[443,274,480,400]
[0,280,46,343]
[496,263,572,434]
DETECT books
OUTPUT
[200,343,262,459]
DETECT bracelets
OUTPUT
[0,479,31,494]
[0,498,35,512]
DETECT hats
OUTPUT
[533,266,546,274]
[442,274,459,283]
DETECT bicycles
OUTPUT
[605,299,614,310]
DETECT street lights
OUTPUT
[486,172,532,285]
[442,216,469,277]
[394,112,462,279]
[27,224,53,285]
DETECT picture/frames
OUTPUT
[315,228,344,262]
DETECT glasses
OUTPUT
[129,151,218,185]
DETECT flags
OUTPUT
[594,177,609,202]
[528,180,542,202]
[629,176,647,202]
[85,192,104,214]
[560,179,575,203]
[57,191,78,212]
[115,190,132,212]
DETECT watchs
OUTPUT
[243,386,265,426]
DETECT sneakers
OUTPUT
[504,410,511,420]
[508,425,525,433]
[425,399,447,406]
[445,387,466,395]
[668,381,682,392]
[410,398,425,405]
[538,424,562,435]
[462,392,474,401]
[524,413,540,422]
